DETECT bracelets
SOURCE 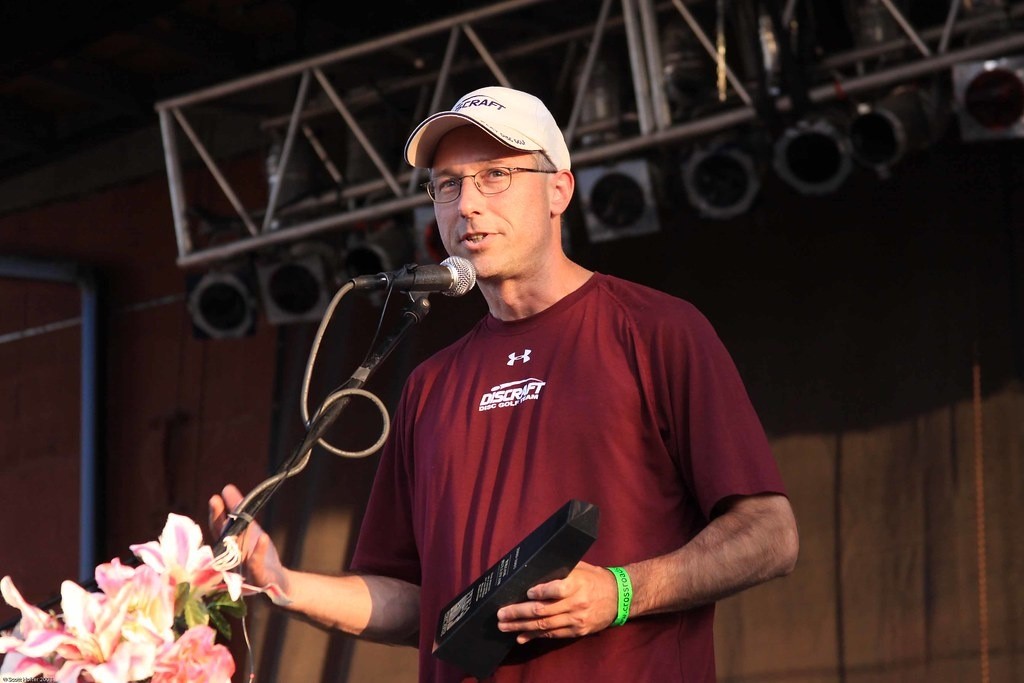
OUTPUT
[607,566,632,628]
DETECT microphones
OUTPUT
[348,256,477,297]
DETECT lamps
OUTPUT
[186,47,1024,345]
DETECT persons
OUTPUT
[209,86,801,683]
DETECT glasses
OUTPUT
[421,168,558,203]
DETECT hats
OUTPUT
[404,86,571,171]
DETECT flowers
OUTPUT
[0,512,290,682]
